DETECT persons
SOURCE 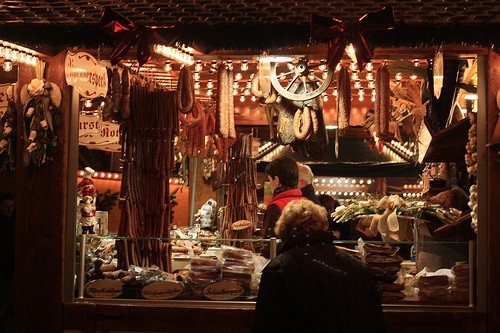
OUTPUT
[0,193,16,333]
[318,193,346,241]
[253,199,386,333]
[297,162,321,205]
[261,156,308,259]
